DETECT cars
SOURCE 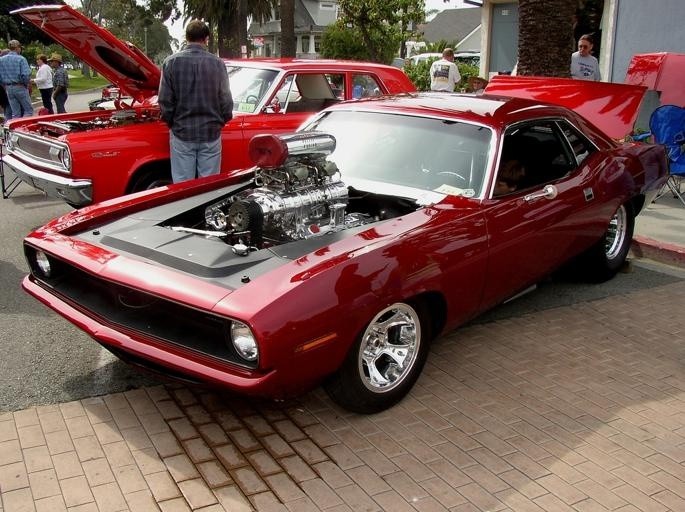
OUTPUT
[0,2,418,216]
[16,72,675,423]
[100,82,129,98]
[86,96,159,111]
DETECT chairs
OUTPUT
[645,102,685,213]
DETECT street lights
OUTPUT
[142,26,148,58]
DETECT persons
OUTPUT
[467,75,484,95]
[570,35,602,82]
[0,39,34,119]
[429,47,461,92]
[47,53,69,113]
[30,54,55,114]
[158,20,233,185]
[493,160,525,196]
[0,48,24,121]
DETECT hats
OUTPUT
[47,53,62,63]
[8,40,25,50]
